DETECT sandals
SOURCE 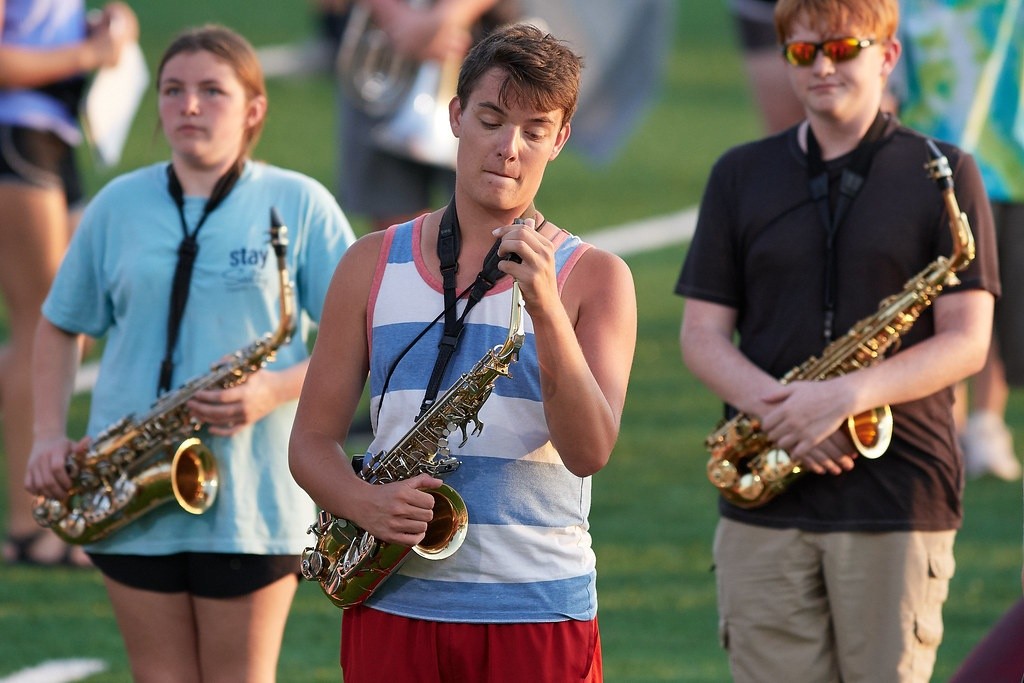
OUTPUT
[6,528,92,577]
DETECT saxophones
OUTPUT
[29,204,297,545]
[704,138,977,511]
[301,218,525,608]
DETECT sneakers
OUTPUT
[959,411,1022,483]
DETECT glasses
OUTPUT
[782,36,889,69]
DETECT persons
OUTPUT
[675,0,1005,683]
[0,0,141,570]
[318,1,524,231]
[286,23,640,682]
[25,30,355,683]
[735,0,1024,485]
